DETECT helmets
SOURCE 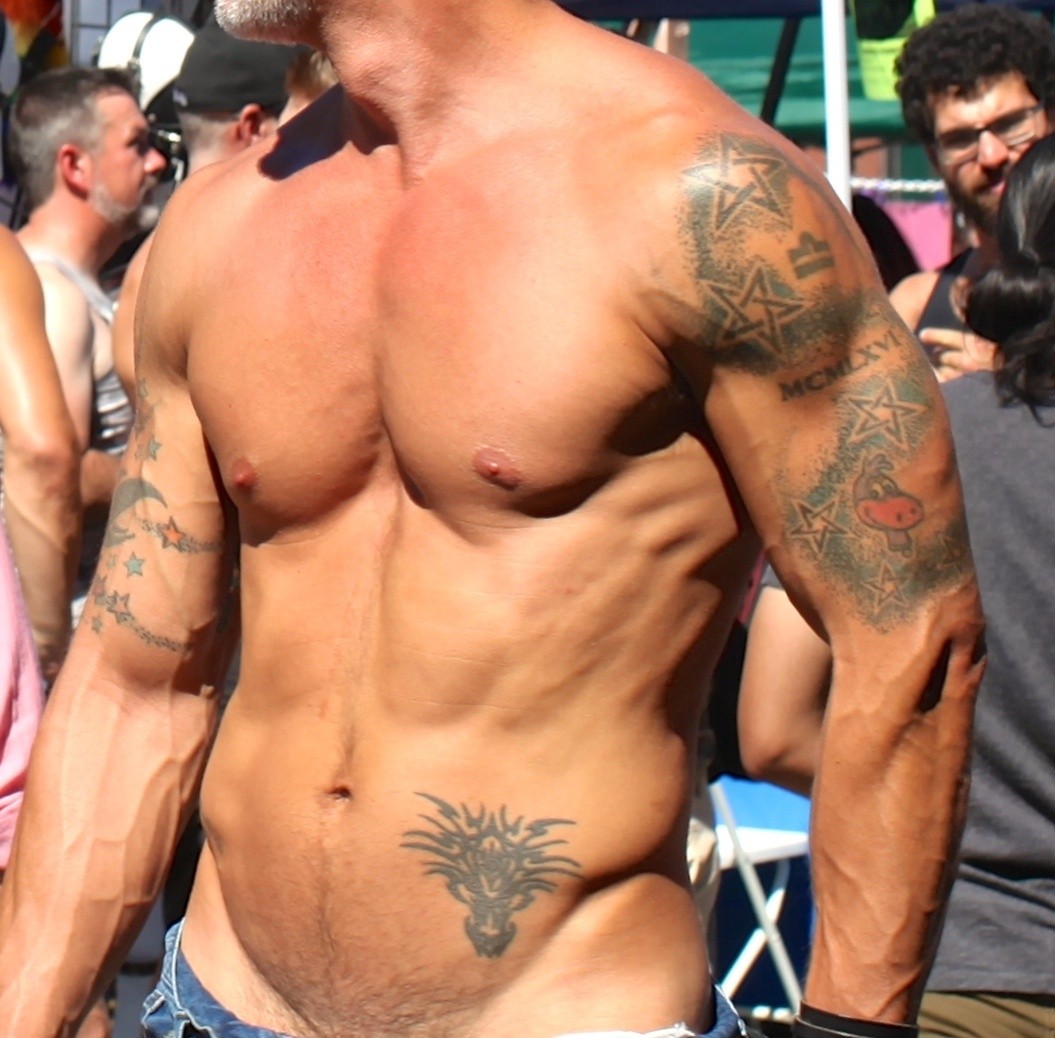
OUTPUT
[96,12,195,114]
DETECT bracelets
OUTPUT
[791,1017,862,1038]
[801,1001,919,1038]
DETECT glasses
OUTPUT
[931,101,1042,165]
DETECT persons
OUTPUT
[6,65,167,630]
[737,132,1055,1038]
[888,0,1055,381]
[0,1,989,1038]
[0,0,1055,1038]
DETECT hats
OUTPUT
[174,25,287,110]
